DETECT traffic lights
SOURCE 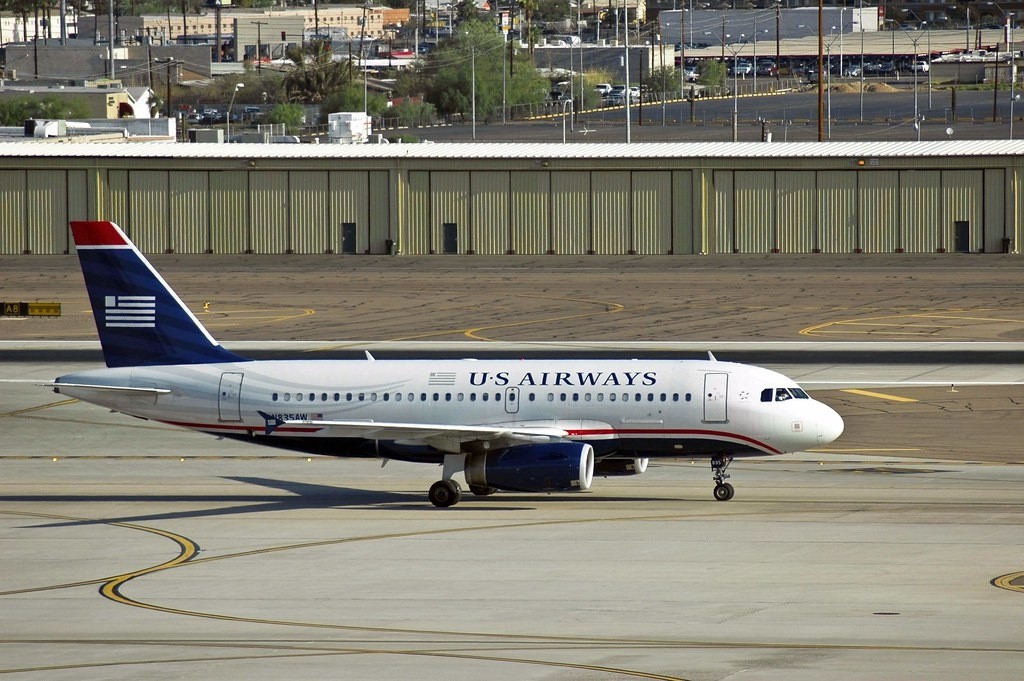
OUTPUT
[281,31,286,41]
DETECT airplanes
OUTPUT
[0,218,848,509]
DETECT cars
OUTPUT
[375,24,458,59]
[531,56,929,106]
[890,22,1022,30]
[159,105,322,125]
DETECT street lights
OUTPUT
[596,8,609,41]
[1009,12,1021,140]
[703,29,769,142]
[902,4,957,110]
[886,17,948,132]
[680,3,710,100]
[540,19,602,101]
[332,29,399,114]
[839,8,847,79]
[839,7,847,76]
[797,21,859,140]
[830,26,837,45]
[226,83,245,143]
